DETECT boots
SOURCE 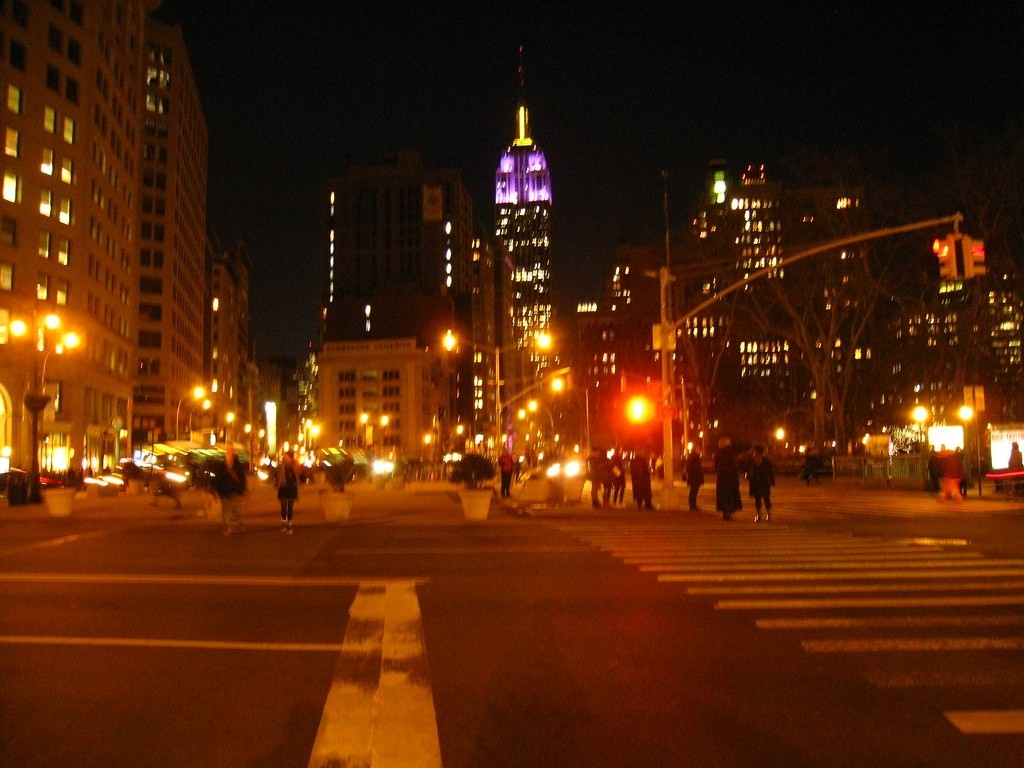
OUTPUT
[286,520,294,535]
[281,519,287,533]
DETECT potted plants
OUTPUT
[39,465,88,518]
[316,459,357,525]
[449,453,496,521]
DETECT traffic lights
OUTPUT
[625,395,653,425]
[934,233,958,280]
[962,235,986,279]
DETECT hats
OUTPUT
[755,446,765,455]
[286,451,295,460]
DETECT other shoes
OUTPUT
[645,505,653,511]
[765,514,772,522]
[637,506,642,511]
[723,514,731,520]
[755,514,761,522]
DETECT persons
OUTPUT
[714,436,743,520]
[587,446,653,511]
[745,445,775,523]
[1009,442,1022,494]
[214,446,247,536]
[686,444,704,510]
[929,443,969,496]
[272,451,300,535]
[802,447,820,485]
[499,448,521,497]
[67,466,93,489]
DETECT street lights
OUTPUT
[188,399,211,441]
[39,332,80,474]
[442,330,553,473]
[175,385,206,441]
